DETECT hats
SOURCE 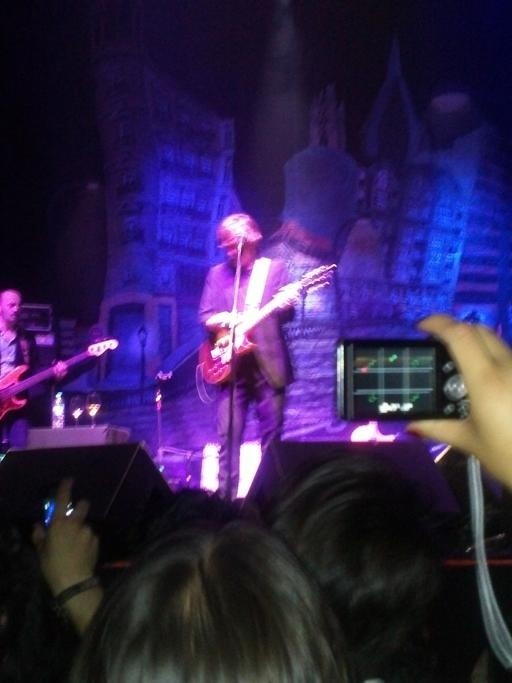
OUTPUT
[215,214,262,248]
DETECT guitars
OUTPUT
[198,264,338,386]
[0,337,119,422]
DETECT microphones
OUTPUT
[237,232,248,255]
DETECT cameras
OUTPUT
[337,337,470,422]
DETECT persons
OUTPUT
[198,212,301,500]
[0,313,510,682]
[1,288,69,450]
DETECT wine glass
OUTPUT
[85,394,101,428]
[70,397,85,429]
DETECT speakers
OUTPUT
[239,440,462,517]
[0,440,176,560]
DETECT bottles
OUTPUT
[51,392,66,430]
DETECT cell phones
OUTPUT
[41,497,74,530]
